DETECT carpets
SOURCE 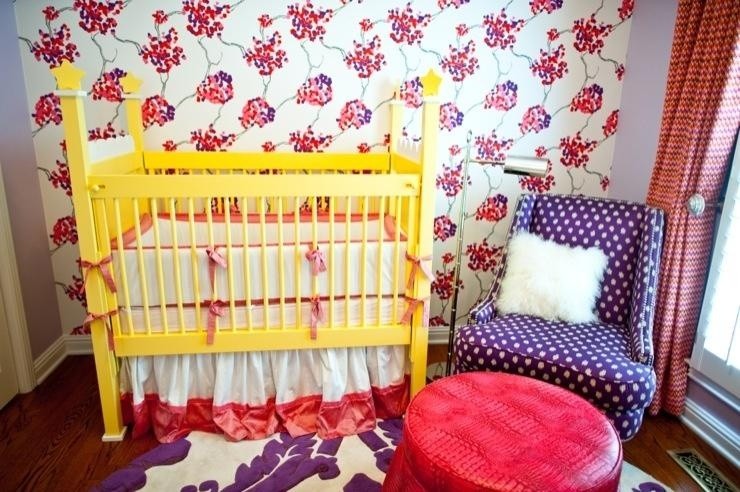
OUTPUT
[93,415,666,492]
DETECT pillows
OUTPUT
[495,232,608,324]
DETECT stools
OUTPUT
[382,372,621,492]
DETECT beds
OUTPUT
[52,61,440,443]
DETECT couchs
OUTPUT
[453,194,664,442]
